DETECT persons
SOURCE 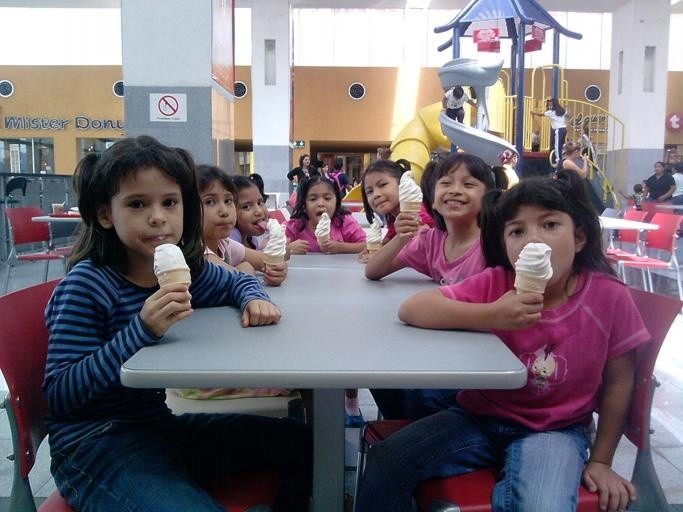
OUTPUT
[166,165,295,401]
[576,130,599,176]
[619,162,683,238]
[442,87,478,124]
[556,143,588,181]
[358,158,436,265]
[225,171,292,262]
[354,168,638,512]
[286,149,360,200]
[530,128,542,152]
[281,174,367,254]
[530,99,571,164]
[364,153,509,287]
[40,135,313,510]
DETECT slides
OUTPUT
[342,102,452,203]
[437,52,520,166]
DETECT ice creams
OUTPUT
[398,170,424,220]
[314,212,331,245]
[263,218,287,271]
[267,217,279,232]
[513,243,554,295]
[365,218,382,254]
[154,243,191,304]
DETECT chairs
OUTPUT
[3,207,77,297]
[598,208,619,254]
[1,276,304,509]
[621,212,683,300]
[353,285,683,511]
[604,207,648,271]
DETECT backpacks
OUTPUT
[331,172,345,193]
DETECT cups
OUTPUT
[52,204,64,215]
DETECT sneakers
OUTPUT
[345,408,364,428]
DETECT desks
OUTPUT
[31,211,83,224]
[118,251,530,511]
[596,215,658,233]
